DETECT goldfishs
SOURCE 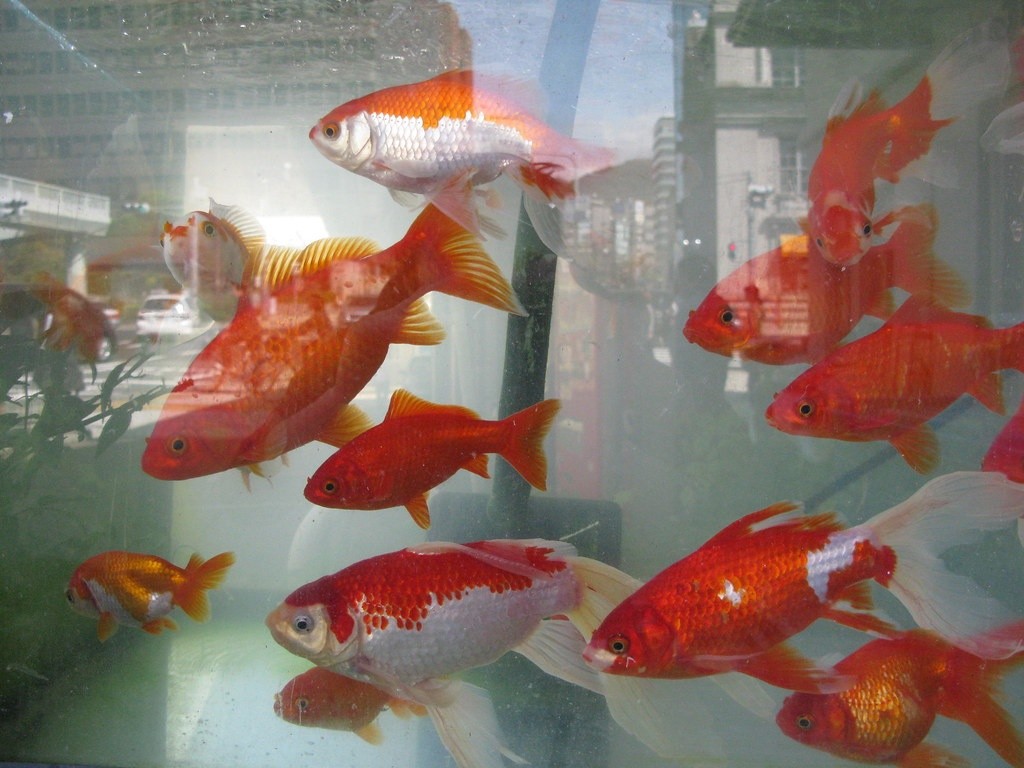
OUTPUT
[36,68,1024,768]
